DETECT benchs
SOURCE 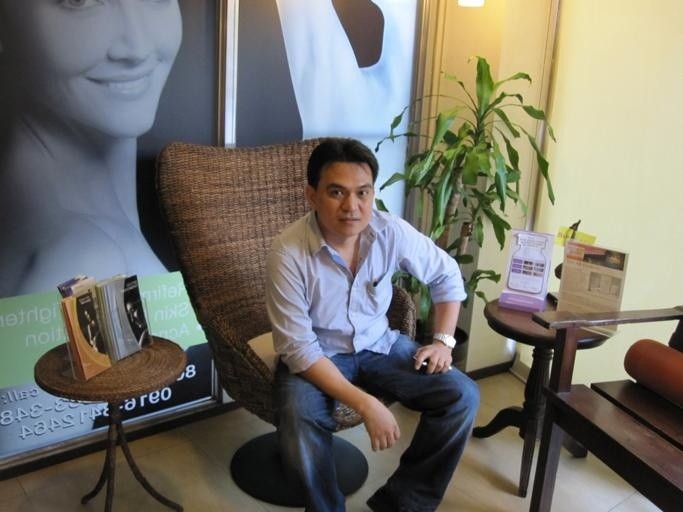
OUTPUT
[530,291,683,512]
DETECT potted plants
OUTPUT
[369,56,552,372]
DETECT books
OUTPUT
[57,274,152,380]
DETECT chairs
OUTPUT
[157,137,416,506]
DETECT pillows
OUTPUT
[624,340,683,407]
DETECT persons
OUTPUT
[0,0,182,297]
[267,139,479,512]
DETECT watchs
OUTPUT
[434,333,457,348]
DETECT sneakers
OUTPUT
[366,483,405,512]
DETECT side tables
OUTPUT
[475,298,617,499]
[35,336,189,511]
